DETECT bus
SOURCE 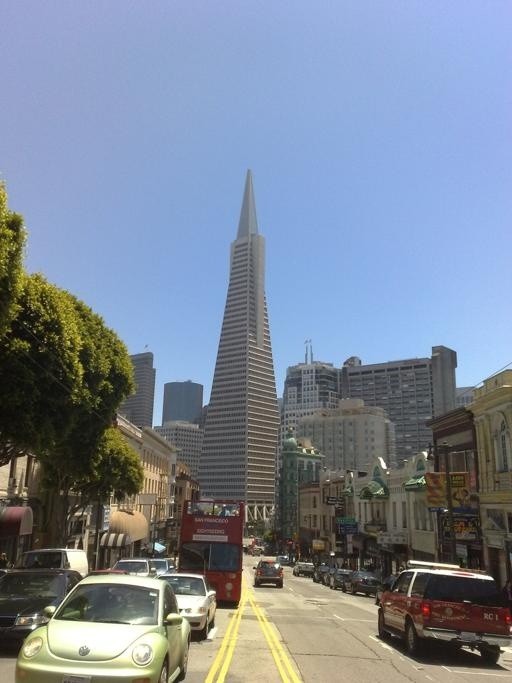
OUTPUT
[177,499,246,609]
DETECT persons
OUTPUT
[218,503,231,516]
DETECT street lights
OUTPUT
[158,473,169,543]
[426,441,458,562]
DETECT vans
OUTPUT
[21,549,88,578]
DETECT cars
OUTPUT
[0,559,217,683]
[253,560,283,588]
[378,560,512,664]
[313,566,398,605]
[252,549,260,557]
[277,556,289,565]
[293,562,314,577]
